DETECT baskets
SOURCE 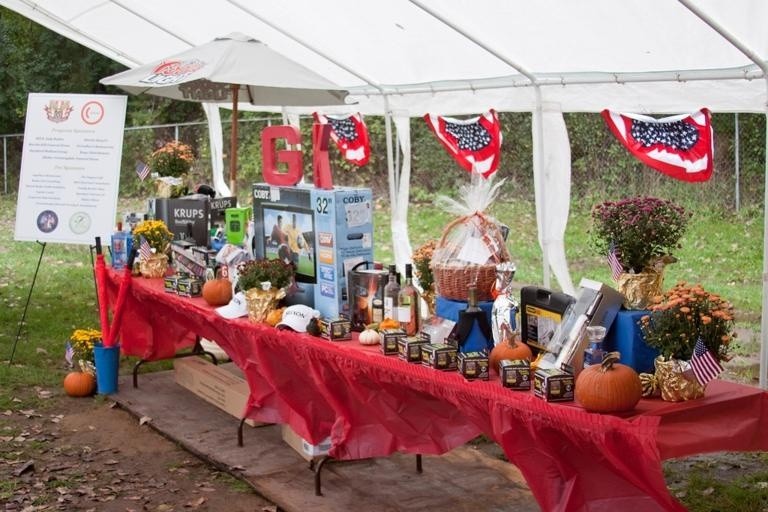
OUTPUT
[431,211,516,301]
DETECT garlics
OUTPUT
[358,328,382,345]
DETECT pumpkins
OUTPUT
[63,371,96,397]
[487,328,532,376]
[574,352,642,415]
[202,268,234,306]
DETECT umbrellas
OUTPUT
[108,244,136,350]
[87,234,112,349]
[99,32,360,193]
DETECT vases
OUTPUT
[79,360,97,376]
[156,177,184,198]
[246,287,287,324]
[422,291,436,315]
[140,255,169,278]
[618,273,665,310]
[653,354,707,402]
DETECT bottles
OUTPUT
[384,264,398,319]
[111,222,129,268]
[398,263,418,336]
[372,275,384,326]
[585,326,607,364]
[465,286,480,314]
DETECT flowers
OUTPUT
[636,280,738,363]
[70,328,103,362]
[238,260,293,290]
[132,220,174,257]
[150,140,196,178]
[412,240,438,288]
[585,196,695,274]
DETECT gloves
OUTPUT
[307,254,313,262]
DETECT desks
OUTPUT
[94,262,762,512]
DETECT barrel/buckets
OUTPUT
[93,342,120,395]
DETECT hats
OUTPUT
[212,289,251,321]
[272,301,321,337]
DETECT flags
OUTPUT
[689,336,724,388]
[600,107,714,184]
[423,109,504,179]
[132,159,152,179]
[310,111,369,166]
